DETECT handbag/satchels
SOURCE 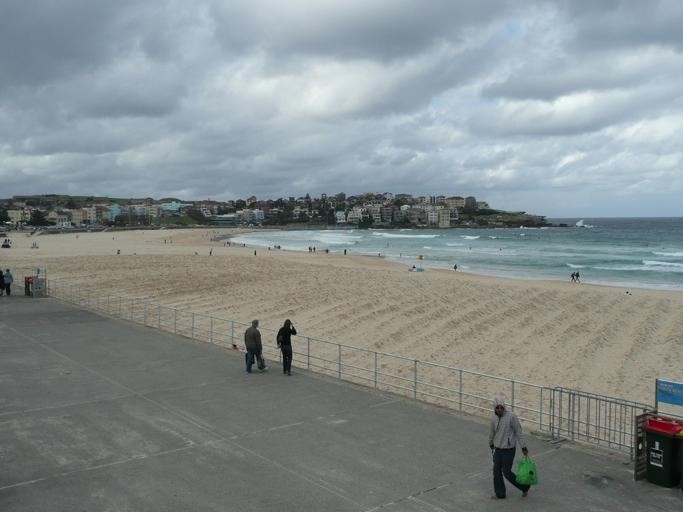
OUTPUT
[245,353,256,364]
[515,453,539,486]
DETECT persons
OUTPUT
[575,271,579,282]
[0,269,5,296]
[488,396,530,499]
[276,318,297,376]
[243,320,268,374]
[569,272,575,281]
[4,268,13,296]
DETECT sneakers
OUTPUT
[490,486,531,500]
[247,365,292,375]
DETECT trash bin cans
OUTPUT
[644,419,682,488]
[25,276,32,297]
